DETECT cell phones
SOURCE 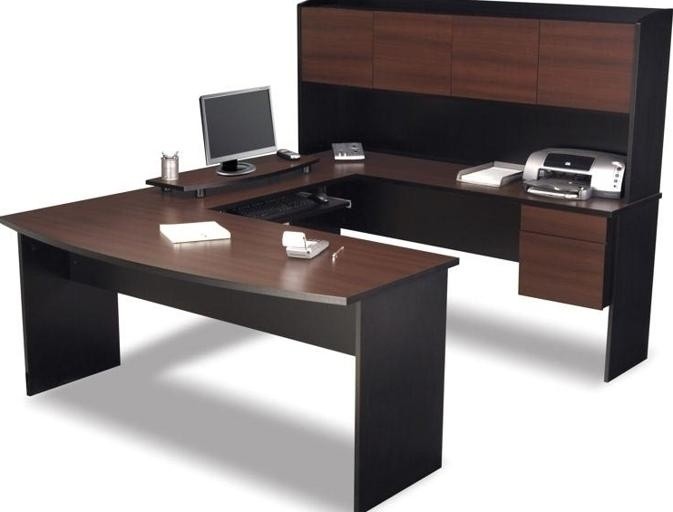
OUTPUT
[277,148,301,160]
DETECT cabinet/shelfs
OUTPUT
[537,19,636,114]
[452,15,539,105]
[300,7,372,89]
[372,9,453,98]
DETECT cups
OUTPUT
[162,157,179,181]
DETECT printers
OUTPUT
[523,147,626,202]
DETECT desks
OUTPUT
[0,151,662,512]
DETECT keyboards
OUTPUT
[230,197,317,221]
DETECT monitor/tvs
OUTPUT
[199,85,277,176]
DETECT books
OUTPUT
[159,219,232,245]
[330,141,365,162]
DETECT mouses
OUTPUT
[312,191,329,203]
